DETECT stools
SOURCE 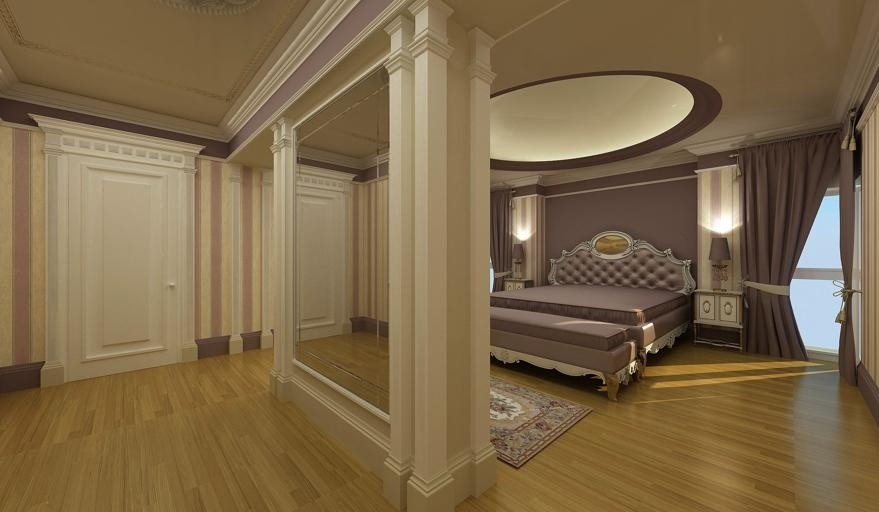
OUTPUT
[489,306,641,403]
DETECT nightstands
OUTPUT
[692,289,746,352]
[504,279,534,292]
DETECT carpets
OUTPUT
[489,374,593,469]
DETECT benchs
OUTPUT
[490,230,696,381]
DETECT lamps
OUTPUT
[509,191,516,209]
[729,154,741,182]
[841,110,857,152]
[511,243,525,280]
[708,237,731,292]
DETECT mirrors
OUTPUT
[286,61,391,432]
